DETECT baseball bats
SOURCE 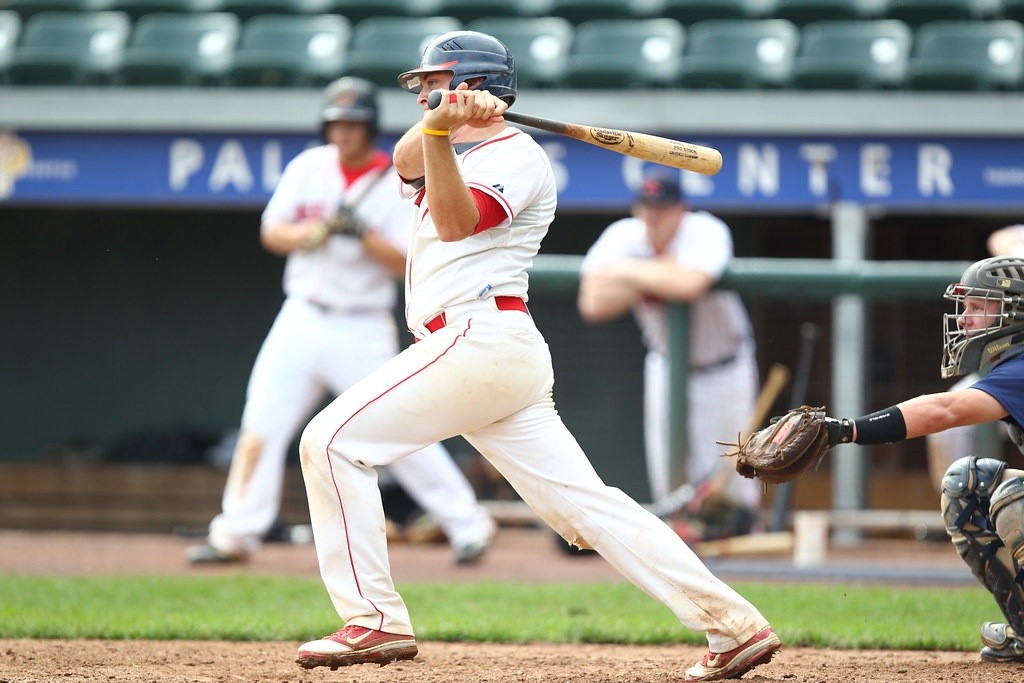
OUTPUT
[427,89,723,177]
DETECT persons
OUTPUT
[737,256,1024,666]
[296,31,781,682]
[185,76,499,563]
[575,175,758,536]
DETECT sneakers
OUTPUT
[687,623,781,682]
[297,621,417,670]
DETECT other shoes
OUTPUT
[978,638,1024,663]
[455,522,497,564]
[191,541,241,564]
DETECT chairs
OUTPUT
[3,0,1024,90]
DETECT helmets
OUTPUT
[395,30,517,110]
[320,76,377,137]
[940,254,1024,381]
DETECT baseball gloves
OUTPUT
[715,403,830,497]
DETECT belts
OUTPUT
[414,298,529,344]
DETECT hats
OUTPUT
[633,163,692,205]
